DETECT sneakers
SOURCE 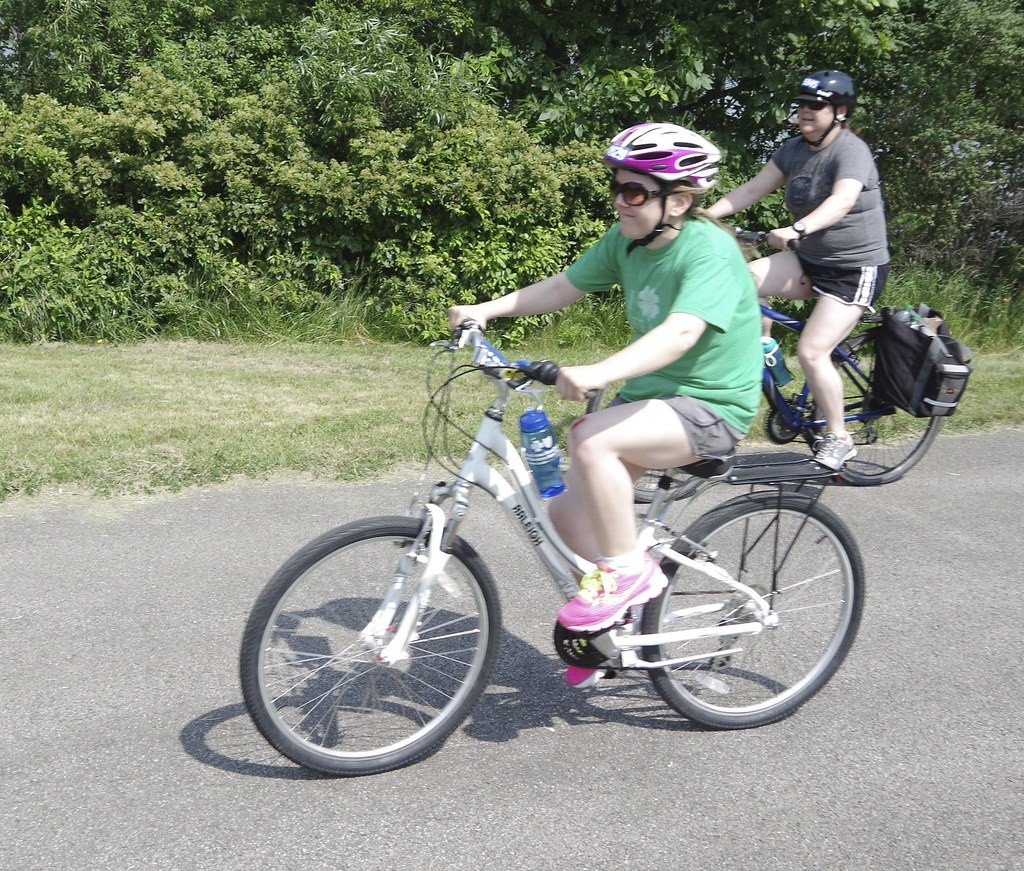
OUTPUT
[558,550,669,632]
[815,431,858,472]
[565,664,608,688]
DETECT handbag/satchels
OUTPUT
[871,305,974,419]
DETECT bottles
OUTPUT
[762,337,791,384]
[520,410,566,499]
[894,304,913,324]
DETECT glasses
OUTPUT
[798,101,835,110]
[610,177,684,206]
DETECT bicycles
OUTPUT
[239,318,867,778]
[586,218,973,487]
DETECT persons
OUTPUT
[702,70,890,470]
[448,123,764,688]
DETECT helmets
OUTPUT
[604,122,722,207]
[793,70,857,119]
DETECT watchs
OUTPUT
[793,220,806,239]
